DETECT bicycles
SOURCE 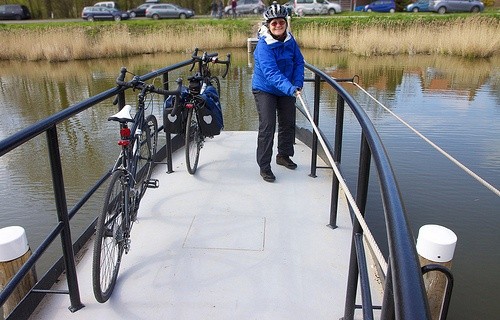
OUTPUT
[93,67,184,304]
[182,47,232,174]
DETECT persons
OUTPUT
[250,4,305,182]
[212,1,217,16]
[232,0,237,16]
[218,1,223,17]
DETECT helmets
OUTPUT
[264,4,288,20]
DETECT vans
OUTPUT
[93,2,119,10]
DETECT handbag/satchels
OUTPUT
[193,86,223,137]
[163,86,192,134]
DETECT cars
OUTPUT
[356,0,396,14]
[427,0,484,14]
[126,0,160,18]
[145,3,195,20]
[406,0,431,13]
[223,0,265,15]
[281,0,342,17]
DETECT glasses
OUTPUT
[269,21,286,26]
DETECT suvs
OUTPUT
[0,4,31,20]
[82,6,129,22]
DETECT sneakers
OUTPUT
[276,154,297,169]
[260,168,275,182]
